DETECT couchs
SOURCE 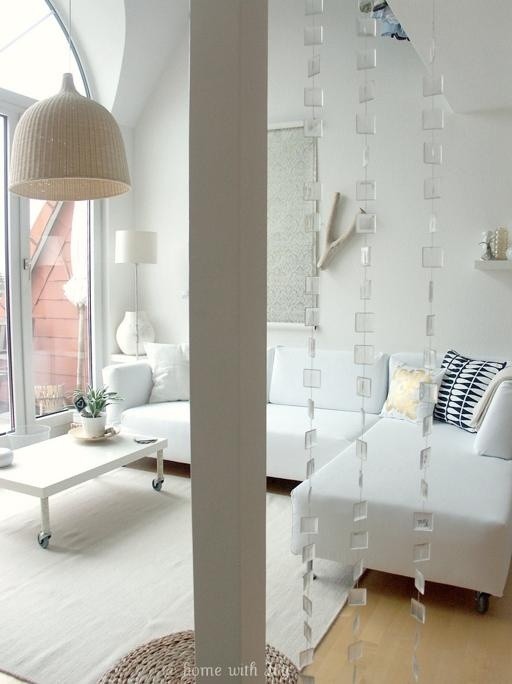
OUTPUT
[99,342,510,617]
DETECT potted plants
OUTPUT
[70,383,126,442]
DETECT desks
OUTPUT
[0,428,171,550]
[108,350,155,374]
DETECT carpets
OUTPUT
[0,461,371,682]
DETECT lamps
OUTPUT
[6,0,130,199]
[114,228,162,362]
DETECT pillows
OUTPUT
[431,345,509,433]
[141,340,191,404]
[376,348,450,434]
